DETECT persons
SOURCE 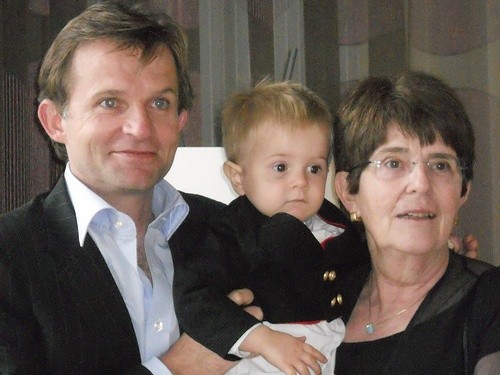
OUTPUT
[330,70,500,375]
[173,74,479,375]
[0,0,263,374]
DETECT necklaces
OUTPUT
[363,269,424,334]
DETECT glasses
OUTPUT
[348,154,469,176]
